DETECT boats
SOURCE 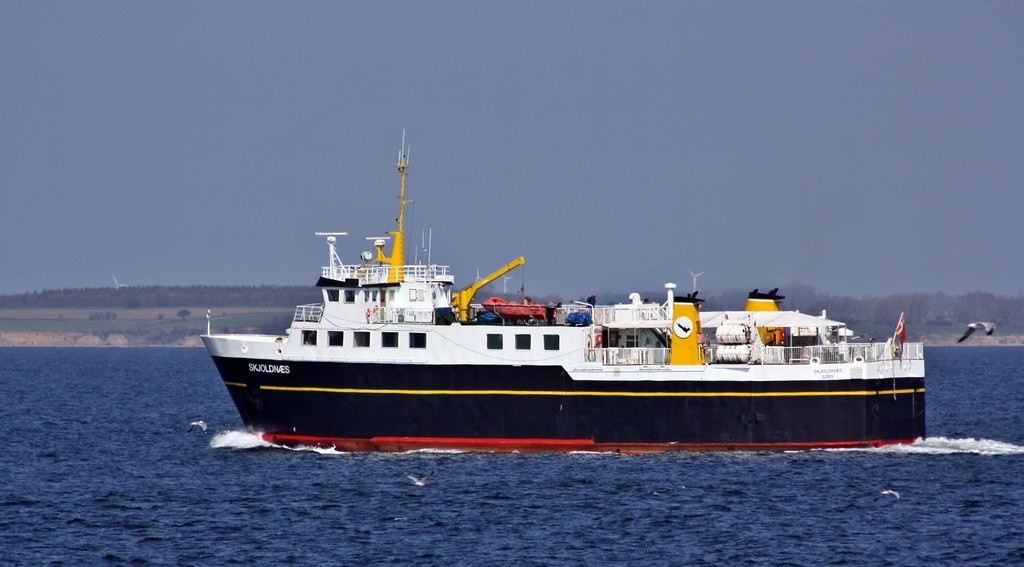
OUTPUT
[197,127,927,455]
[481,297,547,320]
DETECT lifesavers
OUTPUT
[810,357,821,364]
[854,356,864,362]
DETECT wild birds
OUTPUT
[955,320,997,345]
[872,489,902,502]
[186,419,209,434]
[402,469,435,488]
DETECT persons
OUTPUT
[587,294,596,308]
[553,302,562,322]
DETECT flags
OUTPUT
[897,311,905,364]
